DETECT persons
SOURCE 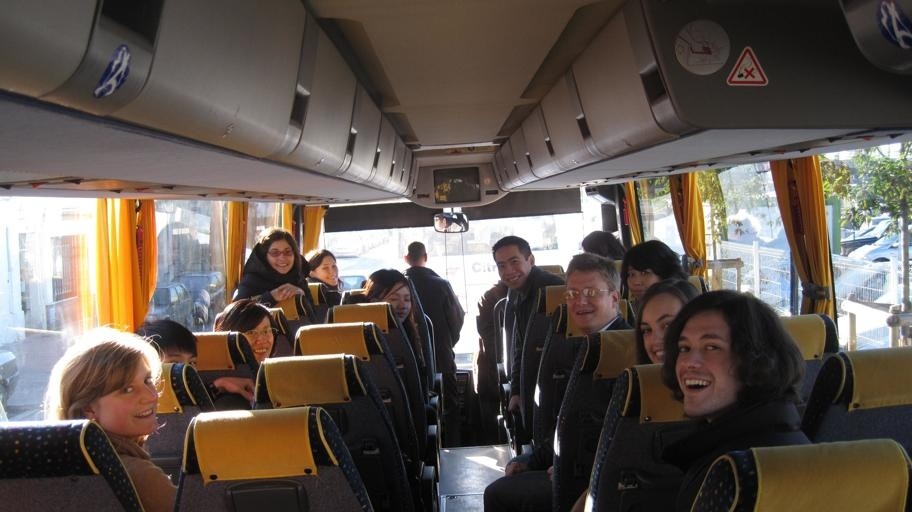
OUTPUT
[136,315,256,408]
[217,299,279,368]
[44,325,179,511]
[621,239,685,299]
[492,235,567,418]
[233,228,316,316]
[660,289,816,512]
[582,229,624,263]
[349,270,421,366]
[305,249,344,291]
[485,253,635,510]
[435,216,448,232]
[636,280,700,362]
[404,242,466,443]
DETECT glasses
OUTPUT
[565,287,613,300]
[267,249,295,256]
[245,327,280,338]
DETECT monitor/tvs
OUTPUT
[432,167,482,203]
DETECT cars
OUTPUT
[0,350,17,403]
[849,224,912,264]
[143,282,194,324]
[170,270,225,318]
[840,220,898,256]
